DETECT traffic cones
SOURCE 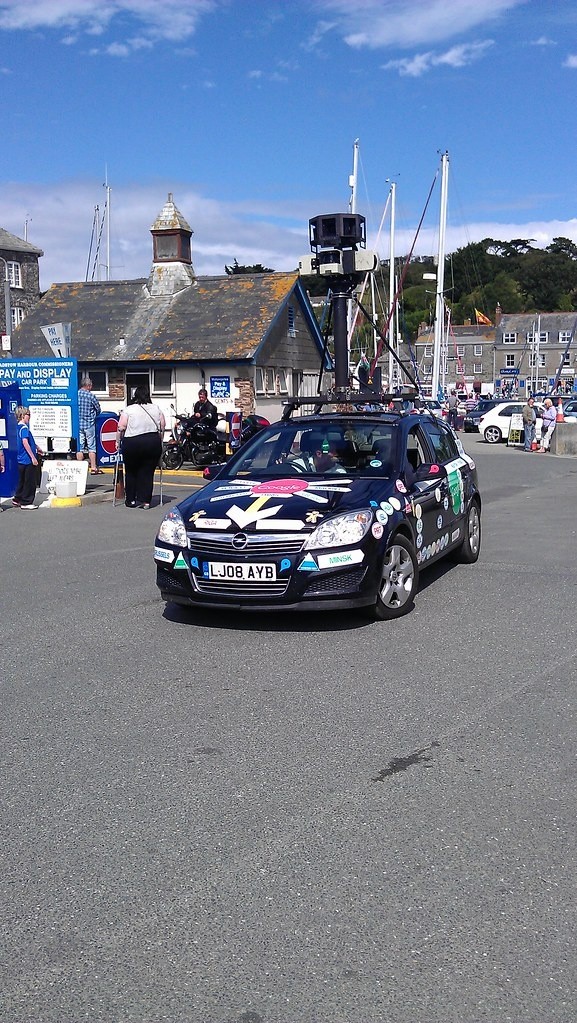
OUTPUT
[556,397,565,424]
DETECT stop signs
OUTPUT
[100,418,118,455]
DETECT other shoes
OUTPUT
[455,429,460,431]
[20,504,38,509]
[537,450,546,452]
[125,500,136,507]
[526,450,533,451]
[12,499,20,506]
[140,503,150,509]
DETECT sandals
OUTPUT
[91,468,105,475]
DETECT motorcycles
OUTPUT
[163,403,270,471]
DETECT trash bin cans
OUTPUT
[94,410,124,468]
[113,463,124,499]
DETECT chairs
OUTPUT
[368,438,413,473]
[300,430,329,457]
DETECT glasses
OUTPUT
[25,414,31,416]
[545,404,547,405]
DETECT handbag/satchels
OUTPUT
[541,425,548,432]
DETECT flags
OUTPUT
[475,310,491,325]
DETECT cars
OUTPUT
[389,395,577,443]
[154,393,481,621]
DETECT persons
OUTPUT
[363,404,376,411]
[445,391,461,431]
[501,385,518,400]
[536,399,556,453]
[487,392,492,400]
[10,406,43,509]
[0,442,5,511]
[476,393,482,400]
[551,384,571,395]
[115,385,165,508]
[463,393,478,414]
[275,441,348,473]
[185,389,218,435]
[522,398,536,452]
[75,378,104,474]
